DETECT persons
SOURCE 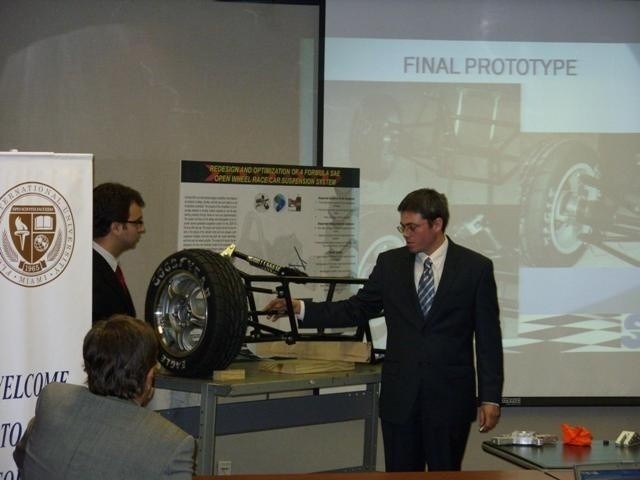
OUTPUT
[92,180,148,324]
[9,313,200,480]
[261,185,505,475]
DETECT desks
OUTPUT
[481,439,640,480]
[152,361,382,475]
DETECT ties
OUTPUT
[418,257,436,318]
[115,265,129,298]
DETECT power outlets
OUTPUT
[218,461,232,475]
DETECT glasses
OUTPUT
[397,221,423,233]
[127,216,143,229]
